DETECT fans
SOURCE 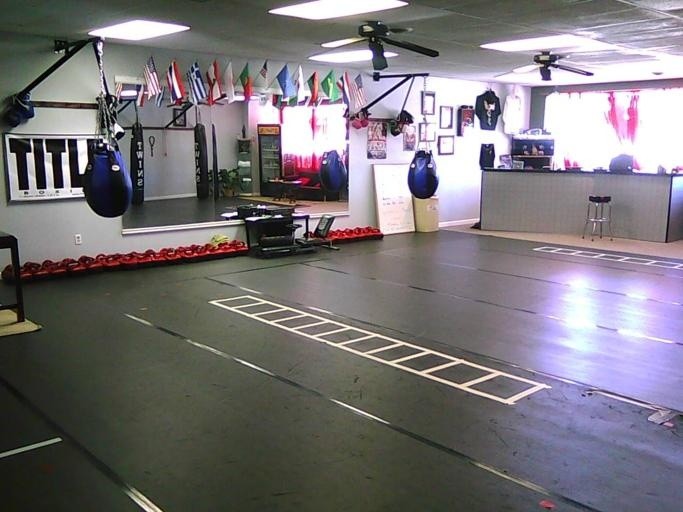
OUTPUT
[319,20,440,59]
[492,51,594,79]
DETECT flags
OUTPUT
[115,83,343,107]
[142,54,368,111]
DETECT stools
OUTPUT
[581,195,614,242]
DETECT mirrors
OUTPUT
[113,75,352,235]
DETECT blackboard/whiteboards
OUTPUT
[373,163,417,236]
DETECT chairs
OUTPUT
[295,214,337,251]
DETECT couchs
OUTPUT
[286,173,339,201]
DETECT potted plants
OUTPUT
[216,167,245,198]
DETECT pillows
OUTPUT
[293,176,321,187]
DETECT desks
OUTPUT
[244,214,309,252]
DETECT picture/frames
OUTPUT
[419,90,454,154]
[173,108,186,126]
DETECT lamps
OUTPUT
[367,40,388,70]
[539,66,551,81]
